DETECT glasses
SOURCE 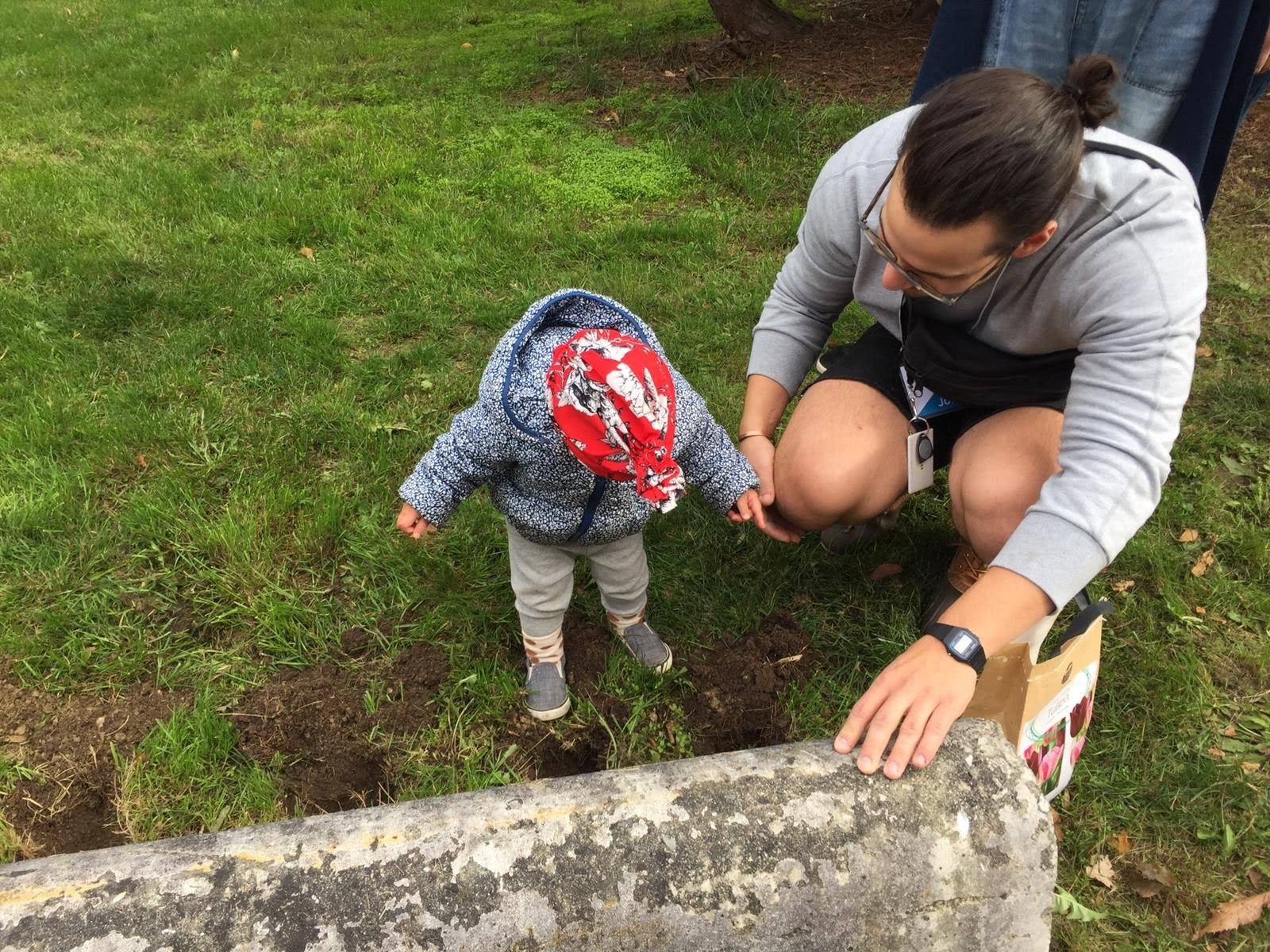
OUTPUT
[857,154,1014,307]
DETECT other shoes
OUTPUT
[919,530,989,639]
[818,478,917,559]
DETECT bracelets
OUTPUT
[738,431,776,447]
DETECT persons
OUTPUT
[738,57,1208,786]
[396,290,766,721]
[910,0,1270,225]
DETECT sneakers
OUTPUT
[610,615,672,673]
[522,652,571,722]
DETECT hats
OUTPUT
[544,329,687,514]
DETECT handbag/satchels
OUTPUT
[961,585,1114,803]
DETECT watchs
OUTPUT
[923,622,987,679]
